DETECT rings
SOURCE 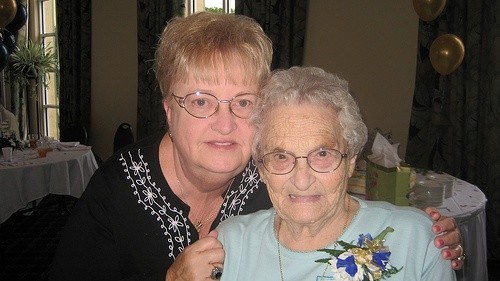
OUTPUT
[211,263,223,280]
[457,256,465,263]
[457,244,464,256]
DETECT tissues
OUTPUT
[365,132,412,206]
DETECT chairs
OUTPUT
[112,123,135,153]
[60,119,87,144]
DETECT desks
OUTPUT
[348,165,489,281]
[0,144,99,240]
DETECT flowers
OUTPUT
[315,226,404,281]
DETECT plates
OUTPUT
[414,174,455,208]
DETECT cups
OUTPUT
[38,142,46,157]
[2,147,12,162]
[29,139,37,150]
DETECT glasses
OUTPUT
[171,91,259,119]
[256,149,348,175]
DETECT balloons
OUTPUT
[430,34,465,75]
[0,0,27,73]
[412,0,446,22]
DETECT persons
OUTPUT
[217,67,456,281]
[47,12,467,281]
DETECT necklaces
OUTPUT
[277,196,350,281]
[175,169,225,231]
